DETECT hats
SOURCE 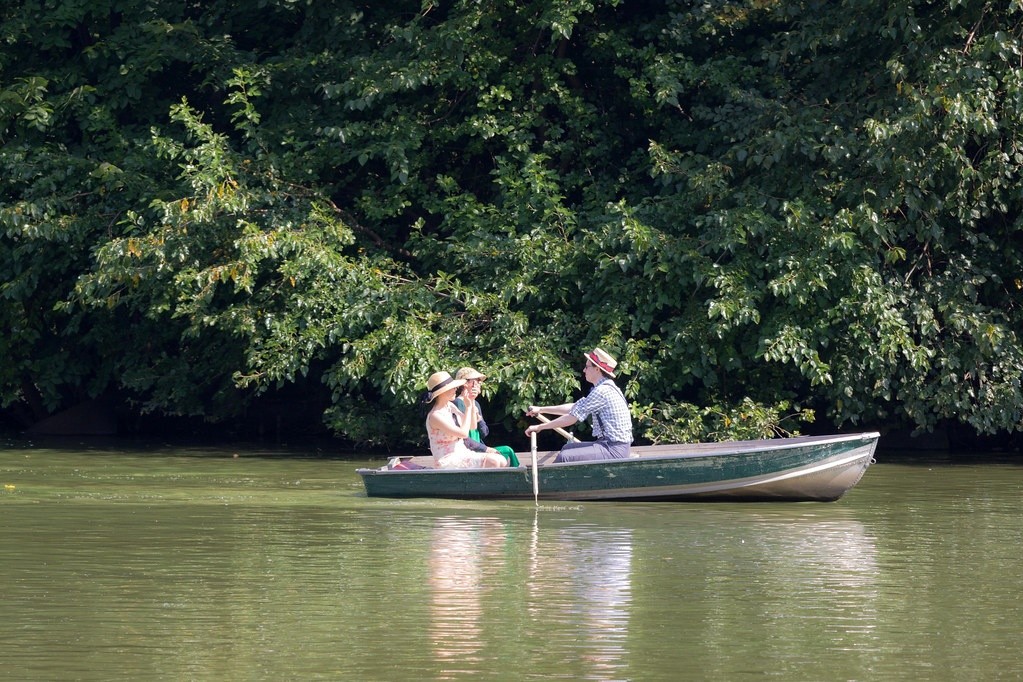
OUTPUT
[455,367,486,379]
[426,372,467,403]
[583,347,617,378]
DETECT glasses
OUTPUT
[585,365,593,369]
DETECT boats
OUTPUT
[354,432,881,502]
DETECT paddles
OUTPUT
[526,405,582,443]
[529,430,540,507]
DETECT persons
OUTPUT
[526,347,634,463]
[453,366,520,467]
[426,371,507,470]
